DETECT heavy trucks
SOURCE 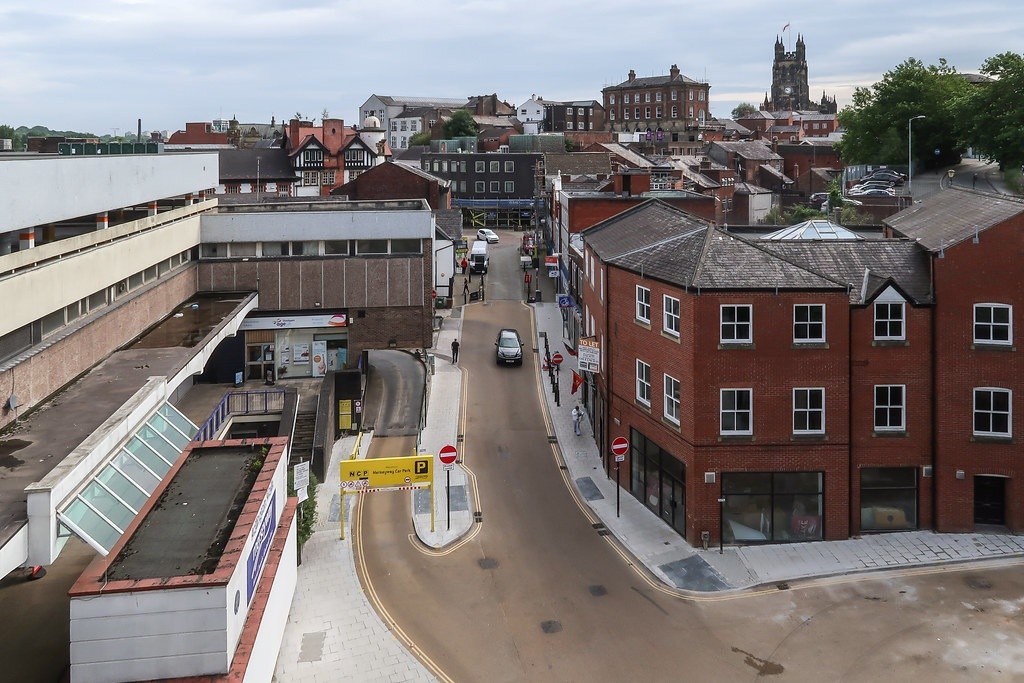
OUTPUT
[467,240,490,274]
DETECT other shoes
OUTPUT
[452,362,454,364]
[574,431,576,432]
[455,361,457,362]
[577,435,580,436]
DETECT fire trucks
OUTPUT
[523,231,539,254]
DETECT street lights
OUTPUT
[909,115,925,195]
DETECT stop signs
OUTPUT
[439,444,458,464]
[553,354,563,365]
[611,436,629,457]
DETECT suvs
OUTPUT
[494,328,525,366]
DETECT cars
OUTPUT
[477,229,499,243]
[846,166,908,194]
[809,193,863,215]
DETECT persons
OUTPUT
[572,406,585,436]
[542,353,549,371]
[973,172,977,189]
[415,349,421,359]
[460,258,467,274]
[450,338,459,364]
[462,278,469,295]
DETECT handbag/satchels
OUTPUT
[578,415,583,423]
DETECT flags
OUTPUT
[570,372,584,395]
[564,344,578,356]
